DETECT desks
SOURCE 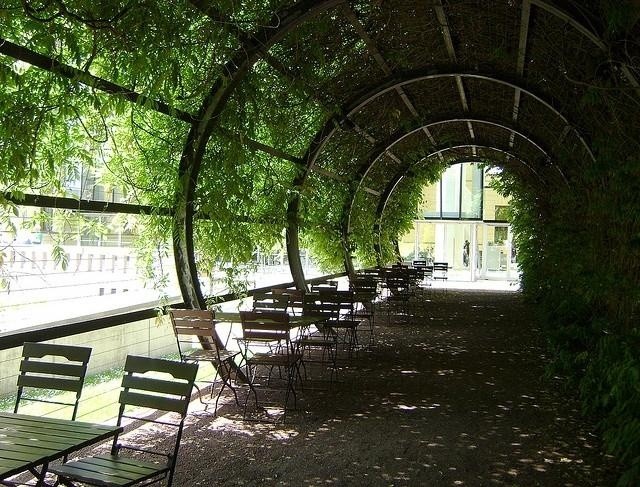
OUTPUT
[0,410,124,487]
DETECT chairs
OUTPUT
[12,341,199,487]
